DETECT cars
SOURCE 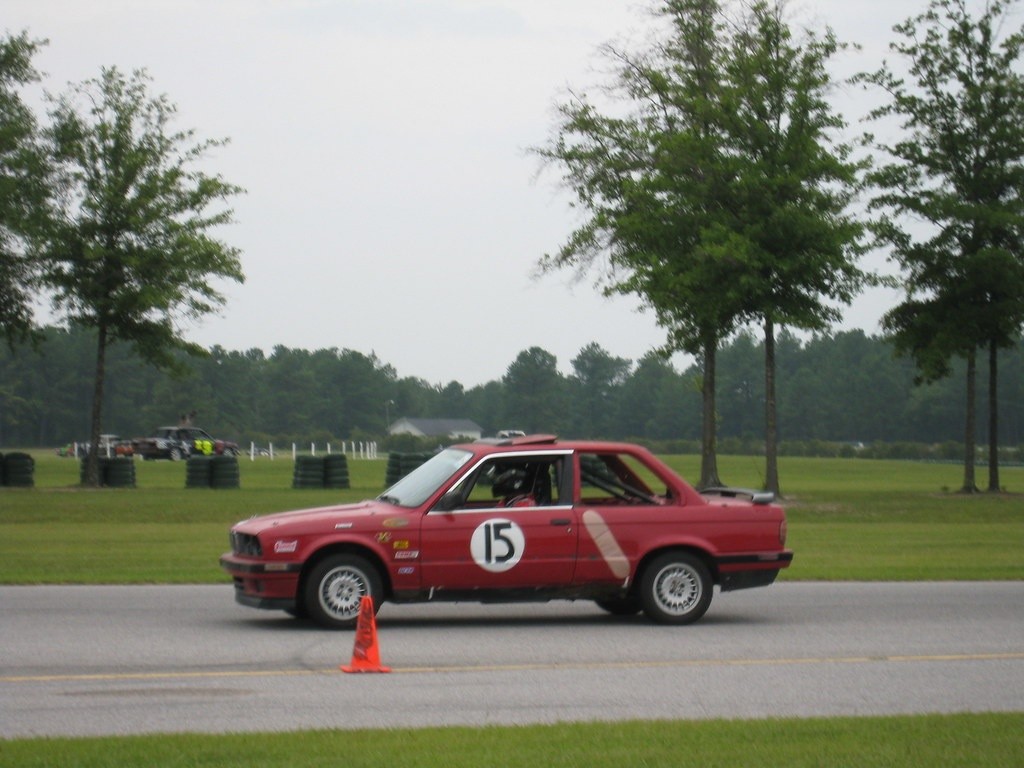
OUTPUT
[131,426,240,462]
[72,434,133,458]
[219,430,795,630]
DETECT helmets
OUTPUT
[486,461,532,498]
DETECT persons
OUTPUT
[487,461,537,508]
[178,409,197,426]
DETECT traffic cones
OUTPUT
[340,595,391,674]
